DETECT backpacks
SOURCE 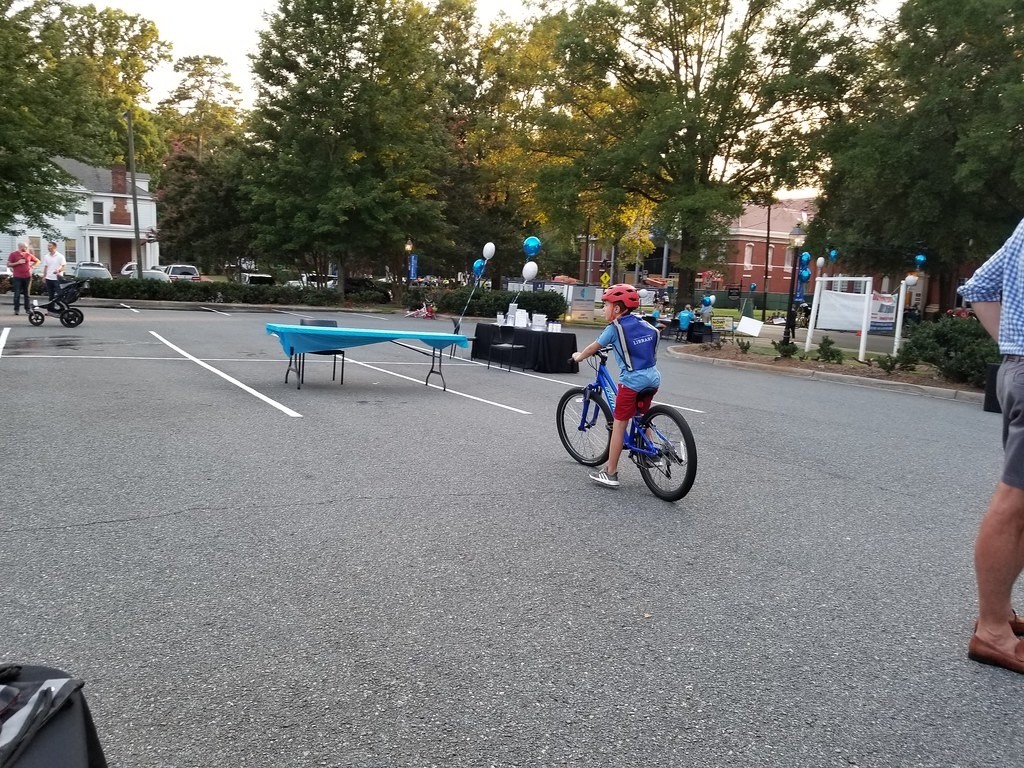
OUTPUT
[605,314,660,372]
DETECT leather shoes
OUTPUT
[968,634,1024,675]
[974,608,1024,634]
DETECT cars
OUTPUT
[164,265,201,282]
[74,267,113,280]
[345,277,394,304]
[129,271,171,282]
[72,261,105,275]
[239,273,276,286]
[0,265,13,278]
[151,266,167,272]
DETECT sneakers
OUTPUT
[646,457,663,467]
[589,466,620,486]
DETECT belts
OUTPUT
[1006,355,1024,362]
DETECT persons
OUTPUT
[957,217,1024,674]
[42,242,67,316]
[572,284,661,487]
[653,292,696,341]
[7,242,40,315]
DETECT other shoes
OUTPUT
[14,311,19,315]
[677,338,686,343]
[26,310,33,314]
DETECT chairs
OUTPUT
[642,316,666,341]
[488,324,524,372]
[450,318,478,361]
[299,319,345,386]
[667,319,712,343]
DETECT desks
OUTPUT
[471,322,579,374]
[266,323,469,391]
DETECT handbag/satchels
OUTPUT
[983,363,1004,414]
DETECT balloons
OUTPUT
[522,236,541,280]
[799,252,811,282]
[817,257,824,268]
[701,295,716,306]
[473,242,495,276]
[750,282,756,291]
[830,250,838,263]
[915,254,926,266]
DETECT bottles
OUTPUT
[548,321,562,333]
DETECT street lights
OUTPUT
[405,233,413,293]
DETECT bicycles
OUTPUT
[556,344,698,502]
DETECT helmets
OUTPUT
[601,283,640,308]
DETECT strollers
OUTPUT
[28,279,91,328]
